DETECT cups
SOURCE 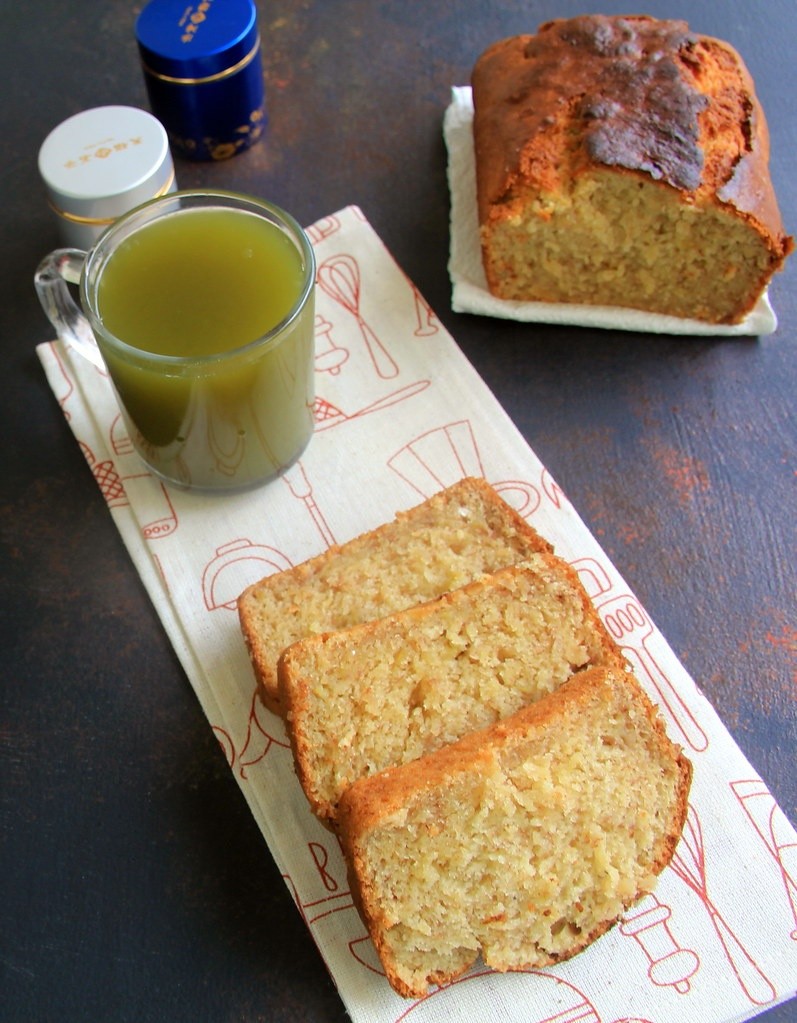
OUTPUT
[34,184,316,495]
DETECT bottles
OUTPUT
[135,0,267,161]
[38,105,176,250]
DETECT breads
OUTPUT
[333,666,694,999]
[238,476,561,708]
[469,15,796,325]
[277,555,629,838]
